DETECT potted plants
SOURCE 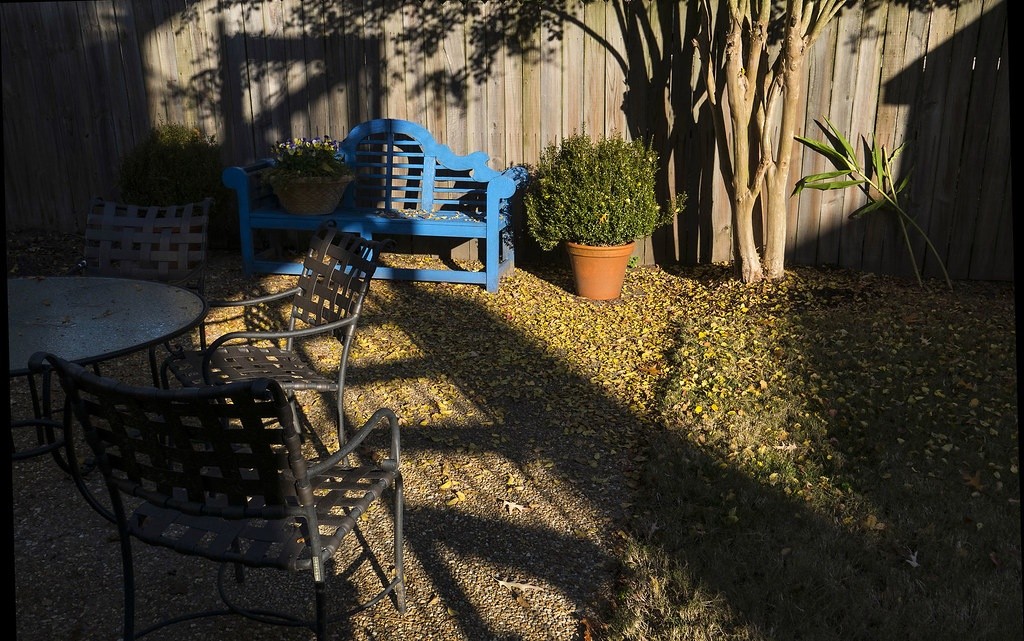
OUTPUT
[522,130,689,298]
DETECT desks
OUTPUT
[9,277,207,483]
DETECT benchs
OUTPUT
[221,118,531,293]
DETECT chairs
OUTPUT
[27,198,405,641]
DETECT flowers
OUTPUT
[257,131,360,181]
